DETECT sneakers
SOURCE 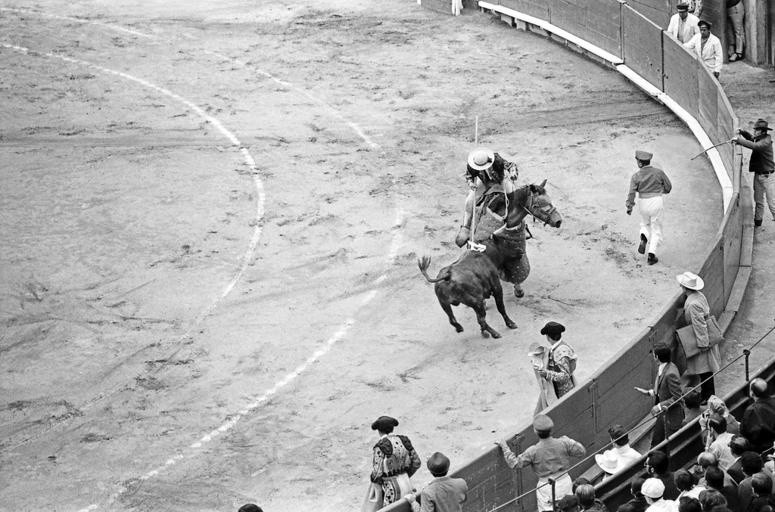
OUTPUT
[637,240,646,254]
[647,253,659,265]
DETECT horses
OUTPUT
[464,176,564,300]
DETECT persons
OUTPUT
[361,416,421,512]
[533,322,577,414]
[731,119,775,225]
[405,452,468,512]
[634,343,685,449]
[667,4,700,51]
[683,20,723,80]
[554,379,775,512]
[494,414,586,512]
[239,504,263,511]
[464,147,518,228]
[727,0,745,62]
[676,272,721,405]
[626,151,671,264]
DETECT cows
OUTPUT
[417,233,523,339]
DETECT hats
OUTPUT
[540,320,565,335]
[675,2,689,12]
[752,117,773,131]
[697,19,712,29]
[371,415,399,430]
[467,146,496,171]
[635,149,653,160]
[426,451,450,475]
[533,413,554,430]
[707,394,729,418]
[595,449,625,474]
[640,477,666,498]
[675,271,704,290]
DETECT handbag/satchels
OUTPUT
[676,315,725,359]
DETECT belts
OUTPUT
[755,171,774,174]
